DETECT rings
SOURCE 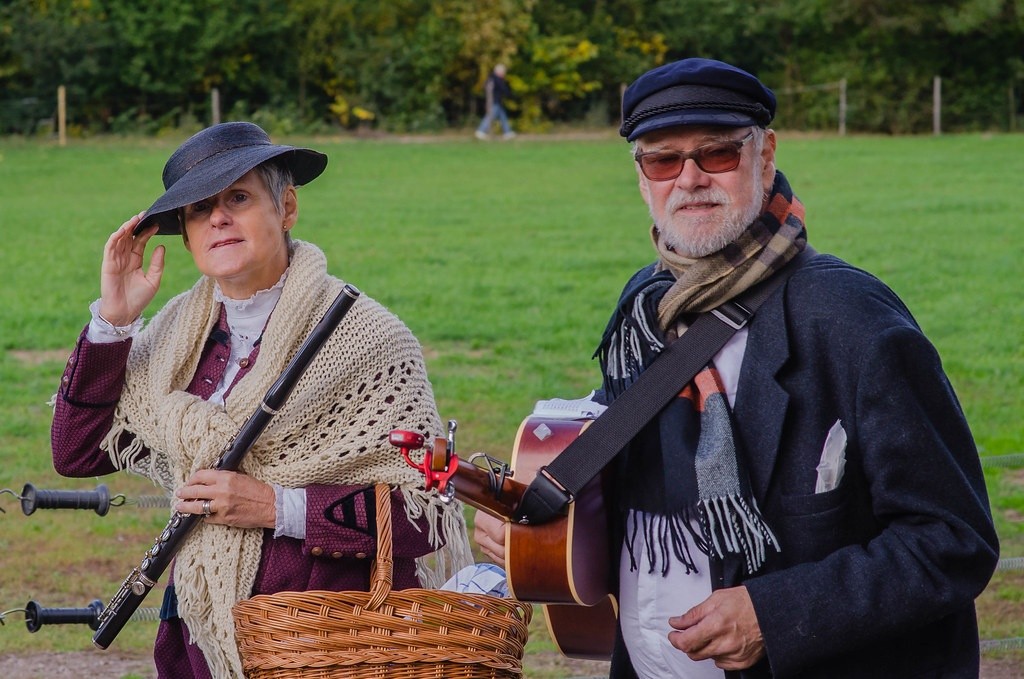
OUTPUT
[201,499,212,515]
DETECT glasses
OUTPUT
[633,130,757,182]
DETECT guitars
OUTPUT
[388,397,621,660]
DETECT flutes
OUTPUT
[89,281,362,651]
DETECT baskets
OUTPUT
[231,483,533,679]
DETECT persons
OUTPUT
[473,62,519,142]
[48,120,475,679]
[472,56,1001,679]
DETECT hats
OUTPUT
[131,120,328,234]
[621,58,777,145]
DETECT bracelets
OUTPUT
[97,311,150,337]
